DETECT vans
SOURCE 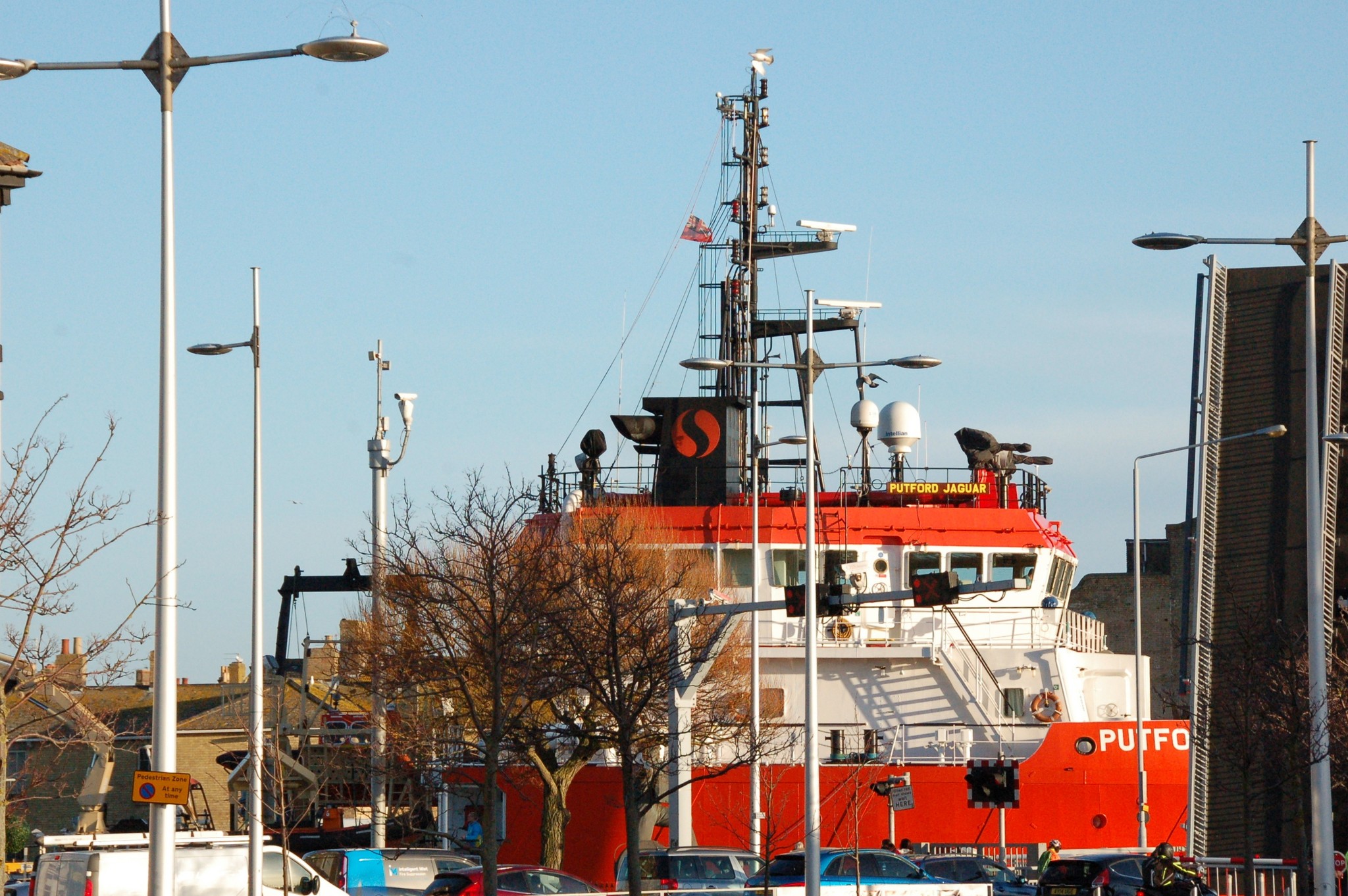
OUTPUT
[302,847,479,896]
[29,828,354,896]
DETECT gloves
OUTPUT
[1190,877,1202,887]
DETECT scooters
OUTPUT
[1136,864,1219,896]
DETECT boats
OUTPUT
[441,46,1191,859]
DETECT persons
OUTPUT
[418,802,435,830]
[794,842,804,850]
[881,839,901,855]
[1153,842,1202,896]
[1047,839,1061,861]
[462,812,483,855]
[898,838,914,854]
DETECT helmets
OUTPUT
[1049,839,1061,851]
[882,839,891,845]
[901,839,911,849]
[1157,843,1173,859]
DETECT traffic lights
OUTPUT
[783,581,824,618]
[910,572,958,608]
[871,781,889,797]
[965,766,1017,802]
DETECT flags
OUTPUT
[680,214,712,242]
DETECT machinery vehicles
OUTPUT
[1,654,116,895]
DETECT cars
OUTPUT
[743,848,962,895]
[1037,846,1197,896]
[422,862,603,895]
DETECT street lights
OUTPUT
[0,0,389,891]
[681,292,943,887]
[1129,427,1293,838]
[186,267,264,896]
[368,338,419,859]
[1127,137,1347,896]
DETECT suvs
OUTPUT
[904,851,1039,896]
[612,845,773,893]
